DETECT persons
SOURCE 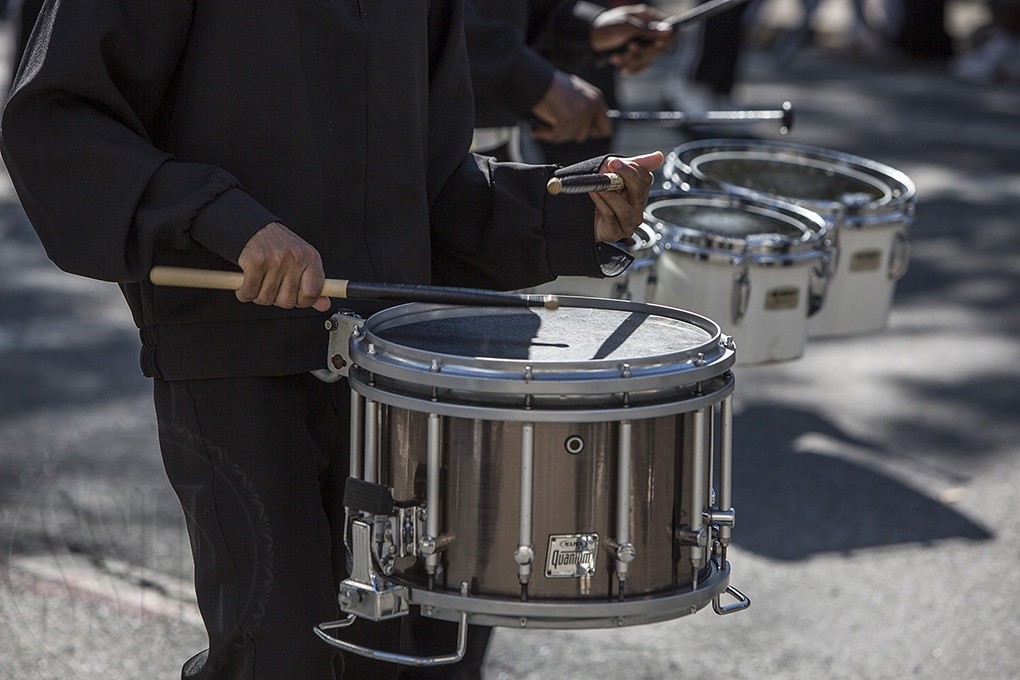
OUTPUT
[1,0,669,680]
[464,1,676,168]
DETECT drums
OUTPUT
[611,195,835,369]
[314,294,749,667]
[664,139,917,340]
